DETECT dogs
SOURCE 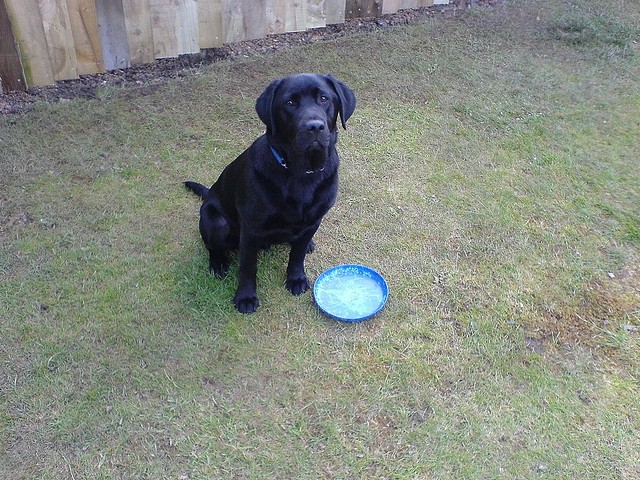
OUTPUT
[183,73,356,314]
